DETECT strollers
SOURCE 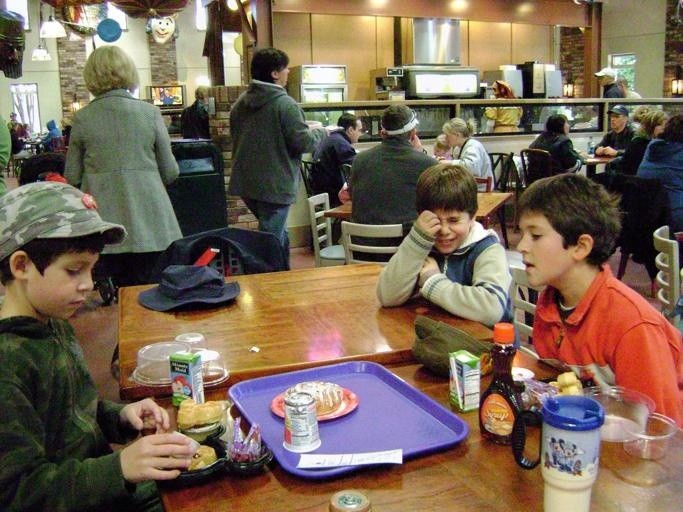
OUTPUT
[92,255,119,304]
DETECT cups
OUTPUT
[283,391,321,452]
[541,395,602,490]
[544,484,592,512]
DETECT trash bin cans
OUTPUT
[166,142,228,238]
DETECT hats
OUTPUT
[413,316,492,377]
[381,111,420,135]
[607,105,628,115]
[0,181,127,262]
[138,265,239,311]
[594,68,616,77]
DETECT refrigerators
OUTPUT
[287,65,347,178]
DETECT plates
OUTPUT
[270,387,357,421]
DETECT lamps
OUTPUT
[564,70,575,98]
[31,0,52,61]
[38,0,67,39]
[671,64,683,98]
[68,31,83,41]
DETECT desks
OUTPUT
[584,157,614,179]
[324,192,513,249]
[27,141,44,154]
[117,264,495,400]
[129,350,683,512]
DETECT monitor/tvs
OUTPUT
[146,84,187,109]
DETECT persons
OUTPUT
[162,89,174,104]
[528,69,683,231]
[1,182,199,511]
[349,103,439,261]
[63,45,185,286]
[432,134,453,161]
[440,117,494,192]
[484,79,522,135]
[516,171,683,429]
[376,164,514,330]
[180,86,211,142]
[0,113,72,196]
[308,112,363,250]
[228,47,330,270]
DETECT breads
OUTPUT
[282,380,342,417]
[189,445,216,470]
[176,399,196,429]
[194,400,223,429]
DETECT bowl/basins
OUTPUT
[159,439,227,482]
[176,331,205,350]
[132,341,191,389]
[196,350,230,387]
[584,385,657,442]
[622,413,679,460]
[226,451,274,474]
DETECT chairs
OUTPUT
[508,267,546,361]
[307,193,346,268]
[51,136,65,151]
[210,242,244,275]
[341,222,403,265]
[476,215,490,229]
[488,153,509,192]
[339,163,354,184]
[673,232,683,303]
[521,149,554,187]
[473,176,492,230]
[494,153,514,248]
[7,155,18,178]
[653,225,681,325]
[301,159,348,252]
[502,152,522,233]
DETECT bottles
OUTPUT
[372,116,378,135]
[330,491,372,512]
[586,135,596,158]
[52,136,65,153]
[479,323,525,445]
